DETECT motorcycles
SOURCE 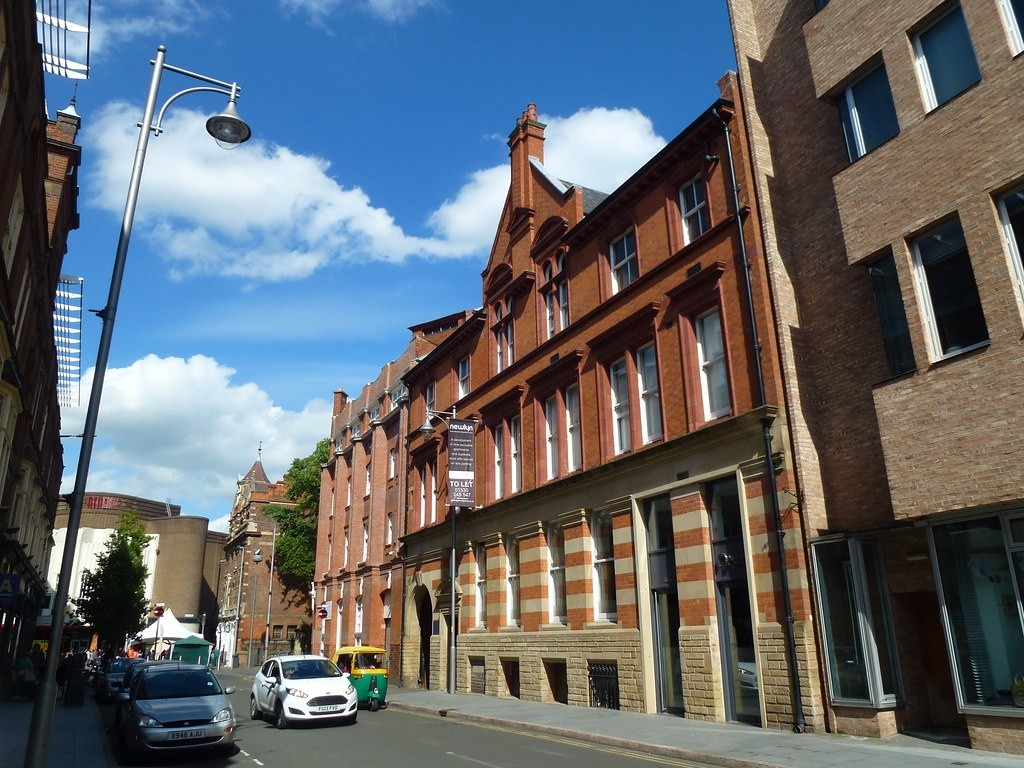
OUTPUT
[331,646,389,710]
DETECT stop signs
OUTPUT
[153,607,164,617]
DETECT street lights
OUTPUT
[23,46,249,768]
[247,549,263,668]
[239,518,277,663]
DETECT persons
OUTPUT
[10,643,170,708]
[364,656,380,668]
[275,664,296,684]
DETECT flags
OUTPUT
[447,417,476,507]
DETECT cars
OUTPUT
[99,658,147,703]
[115,658,190,741]
[124,664,237,760]
[249,653,359,729]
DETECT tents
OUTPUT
[130,607,211,667]
